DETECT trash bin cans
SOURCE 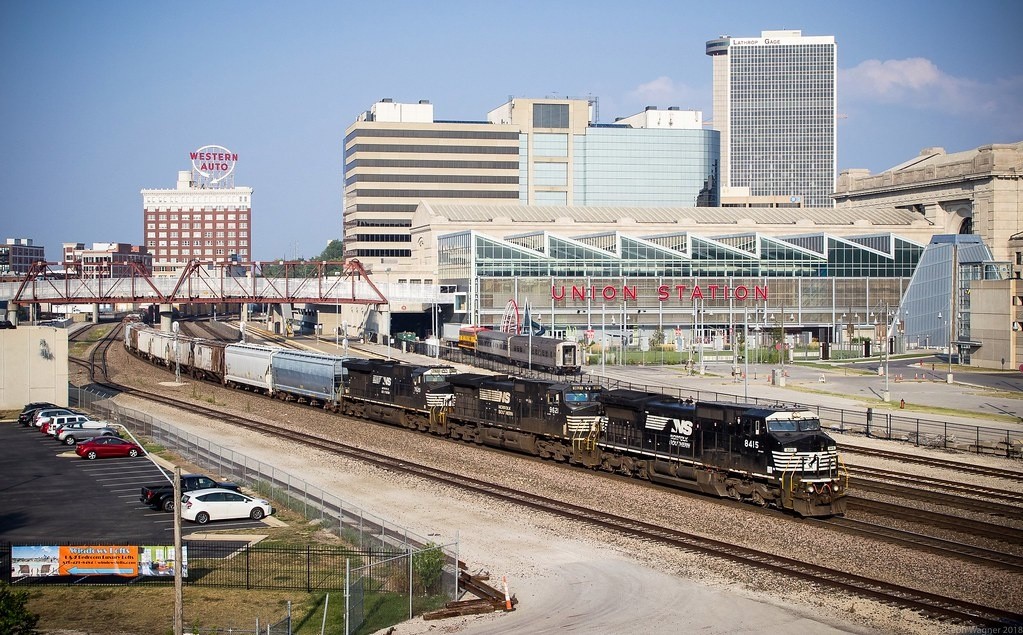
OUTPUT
[878,366,883,375]
[947,374,953,384]
[774,369,786,387]
[586,355,590,366]
[884,390,890,402]
[360,338,363,344]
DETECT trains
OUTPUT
[455,325,583,376]
[124,303,849,522]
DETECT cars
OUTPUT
[75,436,144,459]
[180,488,273,525]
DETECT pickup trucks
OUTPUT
[140,473,242,514]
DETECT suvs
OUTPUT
[54,420,119,445]
[17,402,91,434]
[47,414,109,437]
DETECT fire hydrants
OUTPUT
[901,399,905,409]
